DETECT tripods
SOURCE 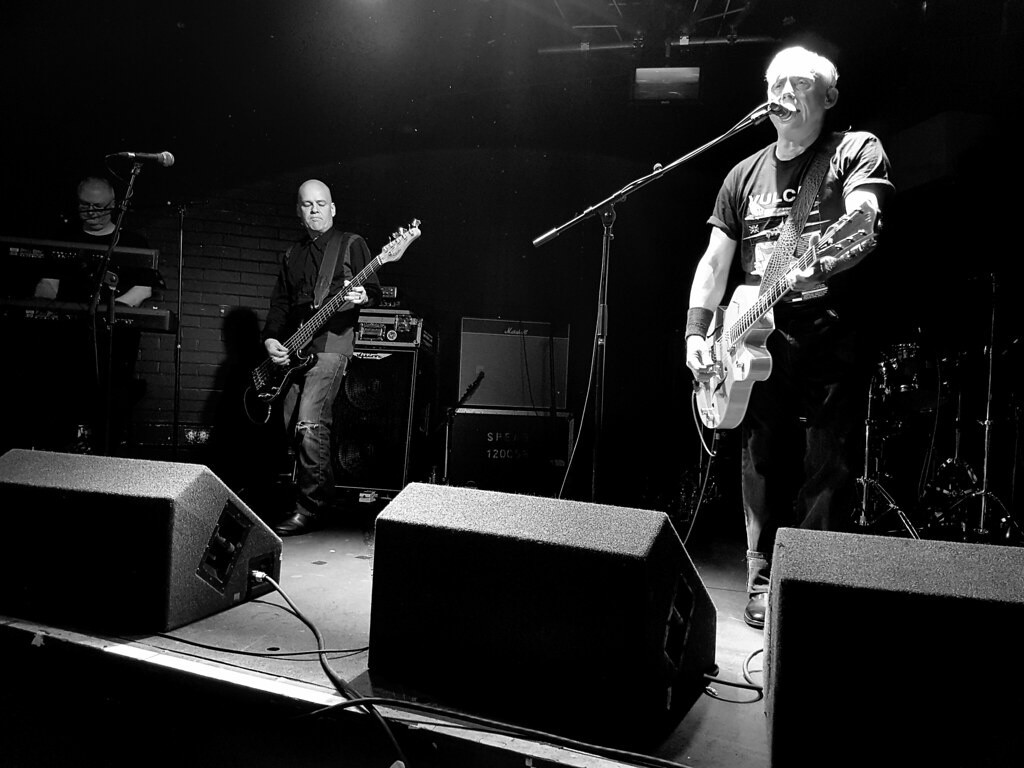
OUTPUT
[847,276,1024,547]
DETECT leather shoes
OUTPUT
[744,593,768,626]
[275,512,308,536]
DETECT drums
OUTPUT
[872,354,923,405]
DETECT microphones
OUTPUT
[770,102,796,122]
[118,151,176,167]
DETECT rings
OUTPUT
[358,295,361,300]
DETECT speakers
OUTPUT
[761,528,1024,768]
[369,482,716,755]
[459,316,571,410]
[330,348,437,492]
[0,448,283,632]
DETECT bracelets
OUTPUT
[685,307,714,341]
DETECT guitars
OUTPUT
[692,198,887,431]
[236,217,423,409]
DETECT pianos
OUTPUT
[0,234,179,461]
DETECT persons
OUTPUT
[262,180,382,536]
[685,46,896,630]
[35,175,153,455]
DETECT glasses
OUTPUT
[78,201,112,211]
